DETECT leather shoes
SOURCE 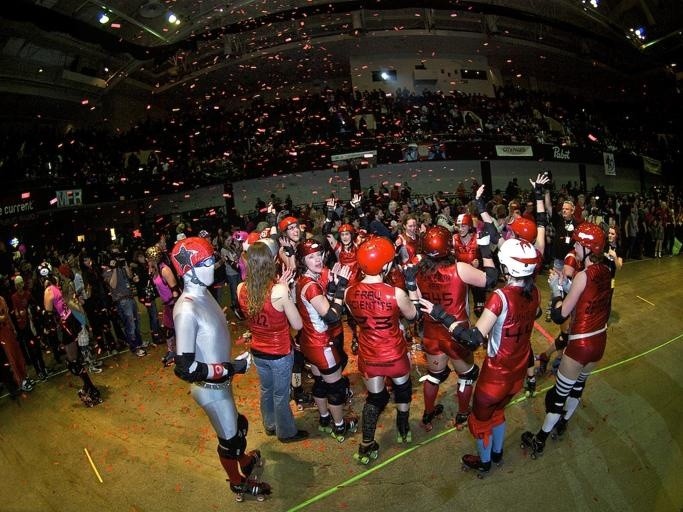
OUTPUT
[277,429,310,443]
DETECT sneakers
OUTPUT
[133,348,147,357]
[89,360,105,374]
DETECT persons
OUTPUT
[1,85,683,500]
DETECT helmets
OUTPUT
[453,214,474,227]
[508,217,539,243]
[355,235,397,276]
[570,220,606,255]
[278,216,300,234]
[295,239,323,258]
[496,237,538,278]
[231,230,248,242]
[169,236,215,278]
[337,222,355,235]
[419,224,452,260]
[142,244,165,263]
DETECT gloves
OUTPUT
[235,351,251,373]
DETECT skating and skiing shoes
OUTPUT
[446,413,470,431]
[229,479,273,502]
[160,350,176,367]
[394,426,413,444]
[77,388,105,407]
[525,353,561,397]
[416,404,446,433]
[242,448,264,478]
[519,418,573,460]
[296,396,318,412]
[461,447,505,475]
[317,416,359,443]
[353,442,380,464]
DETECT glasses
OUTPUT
[193,257,213,268]
